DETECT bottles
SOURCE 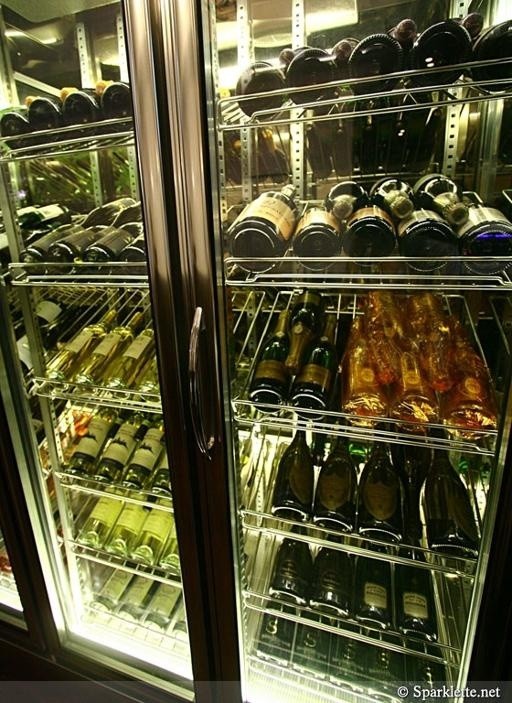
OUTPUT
[7,197,149,275]
[249,290,499,440]
[95,562,187,635]
[0,81,134,154]
[227,173,512,275]
[236,12,512,118]
[16,299,161,402]
[269,525,438,643]
[258,601,446,702]
[78,486,182,576]
[61,408,174,498]
[269,430,480,558]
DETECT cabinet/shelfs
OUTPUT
[1,56,512,703]
